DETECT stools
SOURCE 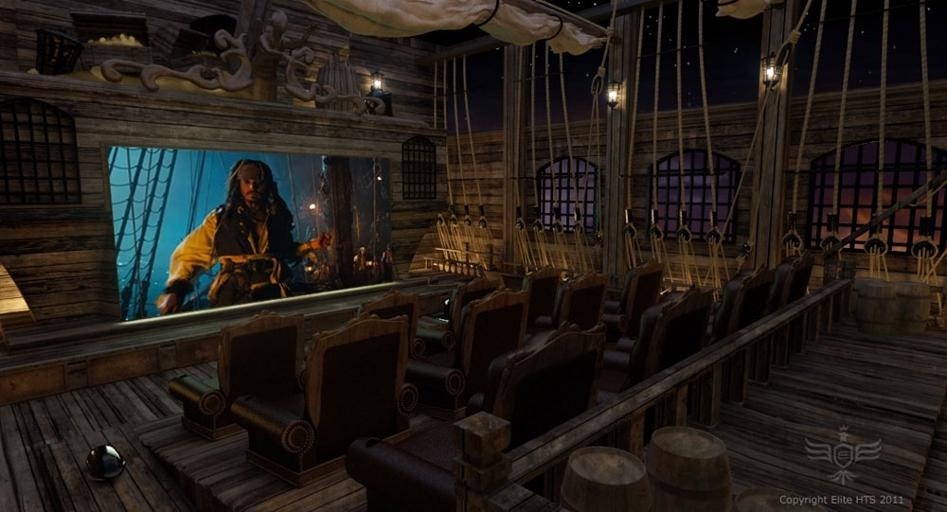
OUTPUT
[847,276,931,337]
[730,485,826,512]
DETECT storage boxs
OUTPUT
[68,6,153,67]
[157,12,237,68]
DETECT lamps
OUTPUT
[606,80,620,110]
[371,71,384,93]
[762,51,780,87]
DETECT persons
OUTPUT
[305,230,395,276]
[154,158,330,316]
[250,9,321,78]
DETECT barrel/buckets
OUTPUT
[731,486,812,512]
[850,276,885,314]
[856,282,894,330]
[893,280,933,337]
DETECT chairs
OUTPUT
[168,248,816,488]
